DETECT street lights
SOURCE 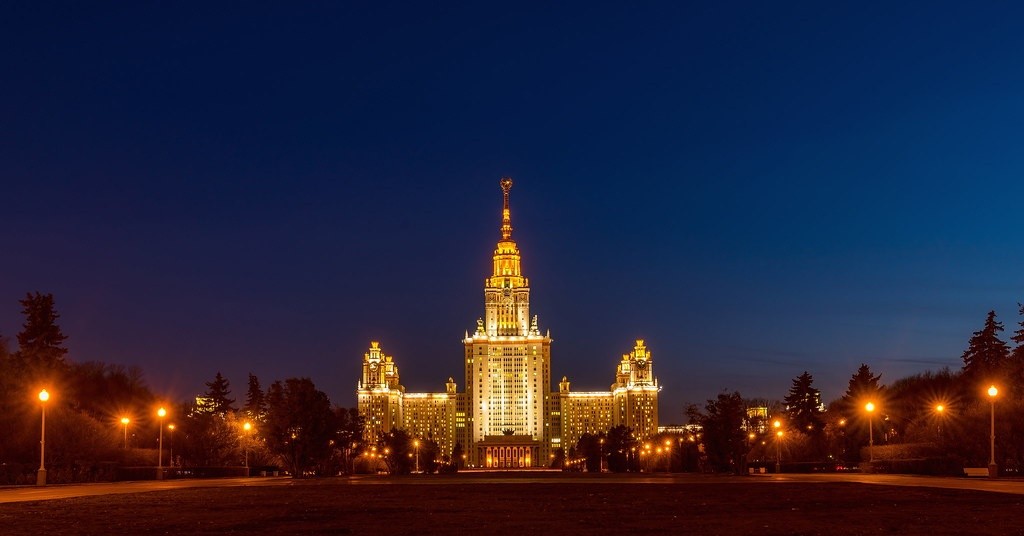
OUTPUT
[774,420,781,473]
[157,407,165,480]
[415,442,418,473]
[866,402,876,474]
[122,418,129,448]
[37,386,49,487]
[601,439,603,472]
[988,385,998,479]
[937,405,943,435]
[245,422,250,478]
[169,425,174,466]
